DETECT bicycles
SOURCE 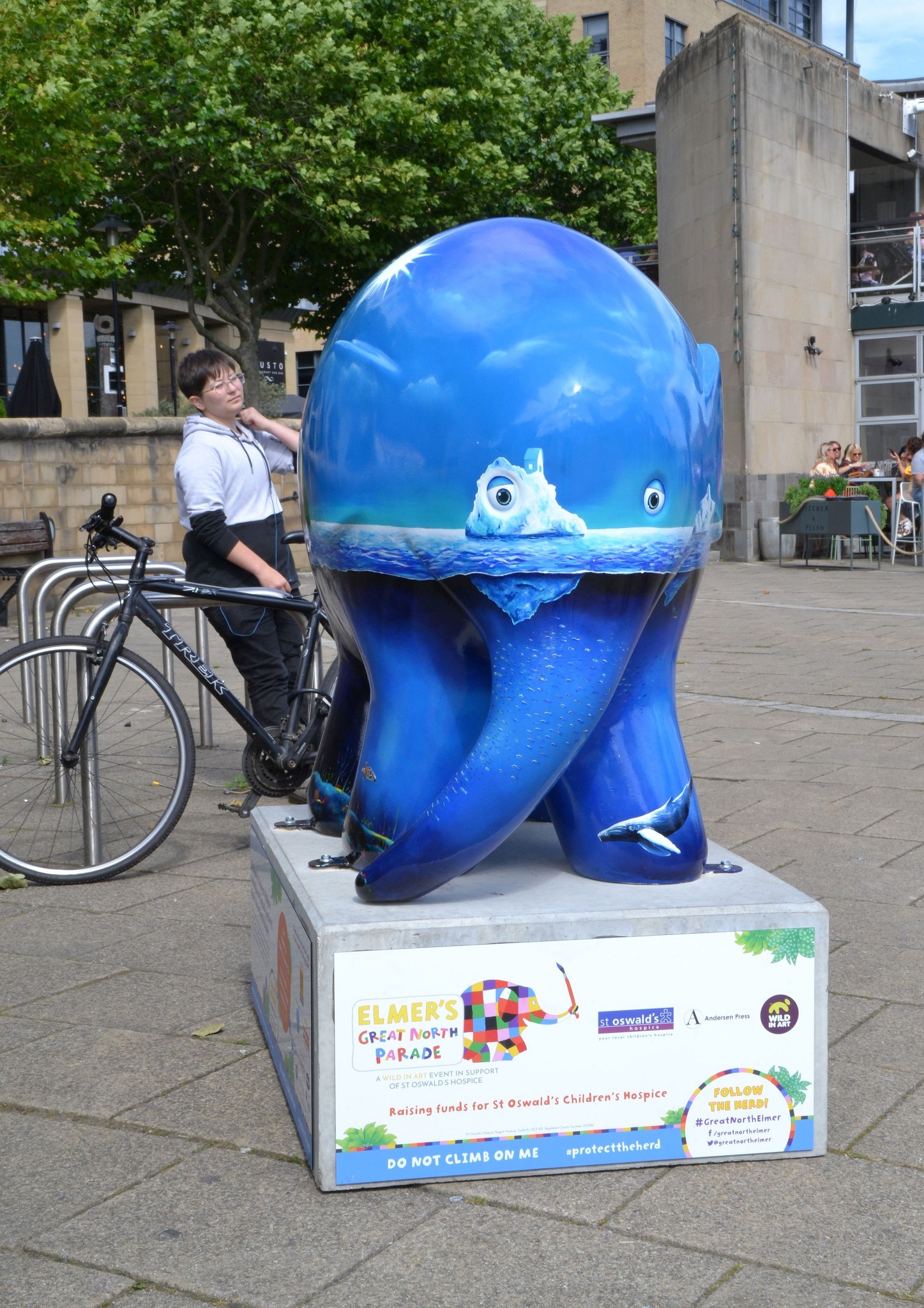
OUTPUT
[0,494,359,886]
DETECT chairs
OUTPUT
[892,480,924,565]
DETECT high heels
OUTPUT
[896,518,918,538]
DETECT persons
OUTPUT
[619,240,658,263]
[175,348,315,804]
[850,213,924,295]
[812,441,869,478]
[885,431,924,540]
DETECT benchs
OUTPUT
[0,511,57,625]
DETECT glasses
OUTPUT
[196,372,245,395]
[852,453,862,457]
[828,447,841,452]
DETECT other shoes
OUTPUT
[288,786,308,804]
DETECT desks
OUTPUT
[843,477,912,557]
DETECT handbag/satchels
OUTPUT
[848,470,873,478]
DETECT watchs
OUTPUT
[849,464,852,469]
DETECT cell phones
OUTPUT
[887,448,894,454]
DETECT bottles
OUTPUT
[873,460,880,477]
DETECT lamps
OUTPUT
[805,336,823,355]
[908,292,916,300]
